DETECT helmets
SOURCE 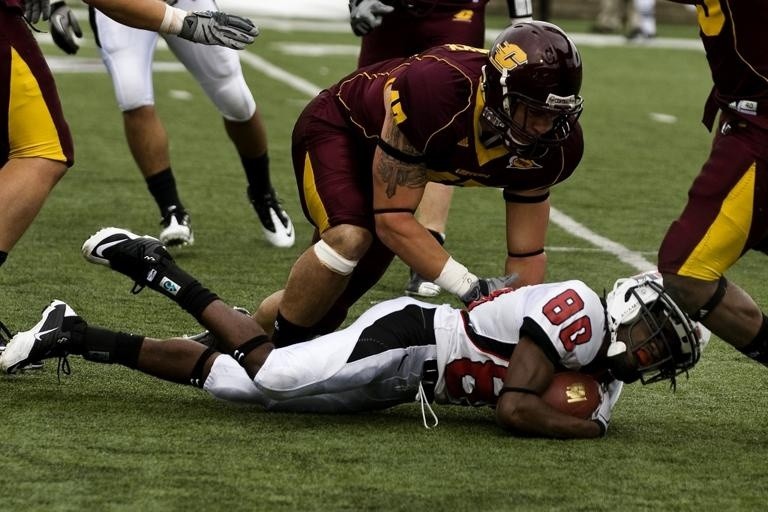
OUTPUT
[606,271,712,386]
[482,21,584,149]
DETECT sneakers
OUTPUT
[81,205,194,294]
[0,299,84,374]
[187,308,251,345]
[247,185,295,248]
[404,269,442,297]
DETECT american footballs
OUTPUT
[540,370,601,419]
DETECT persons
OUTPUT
[591,0,659,38]
[0,226,712,439]
[343,0,535,298]
[46,0,297,251]
[0,0,260,371]
[652,0,768,371]
[189,19,586,358]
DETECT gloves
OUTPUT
[351,0,394,35]
[592,379,623,432]
[461,273,518,307]
[26,0,82,54]
[177,10,259,49]
